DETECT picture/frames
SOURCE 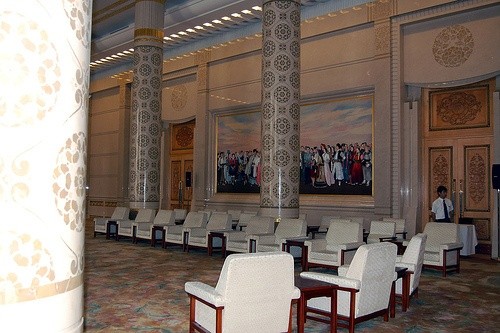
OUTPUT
[214,95,374,199]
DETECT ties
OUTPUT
[443,200,448,223]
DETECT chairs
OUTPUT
[93,206,462,333]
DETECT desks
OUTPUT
[391,266,408,318]
[107,220,118,240]
[294,276,337,333]
[210,231,225,257]
[153,224,165,248]
[285,238,306,271]
[460,223,477,256]
[385,239,409,255]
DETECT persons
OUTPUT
[431,186,453,222]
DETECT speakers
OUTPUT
[492,164,500,189]
